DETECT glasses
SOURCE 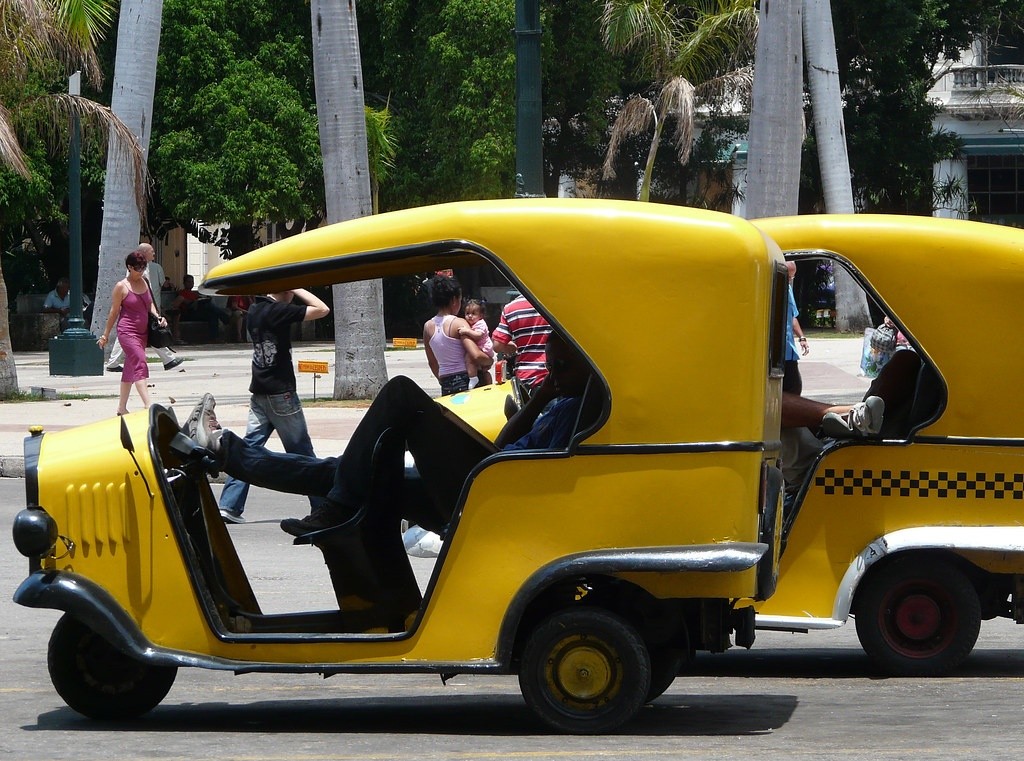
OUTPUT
[131,265,146,272]
[789,275,795,280]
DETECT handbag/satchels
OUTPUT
[148,313,173,348]
[861,328,893,377]
[870,323,895,351]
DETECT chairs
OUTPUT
[172,295,215,344]
[822,350,941,443]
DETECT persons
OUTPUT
[781,393,884,435]
[107,242,184,372]
[185,328,589,536]
[171,275,238,343]
[217,289,329,523]
[492,296,562,424]
[41,278,70,335]
[227,296,253,343]
[783,261,809,395]
[97,252,168,416]
[884,315,916,354]
[424,275,495,396]
[457,298,494,388]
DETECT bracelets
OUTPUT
[799,336,806,342]
[100,334,108,343]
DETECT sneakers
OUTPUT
[281,501,362,555]
[847,396,884,435]
[219,509,246,524]
[182,392,224,453]
[822,413,854,438]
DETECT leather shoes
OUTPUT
[164,358,183,370]
[107,366,123,372]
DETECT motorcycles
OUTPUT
[11,193,784,731]
[424,213,1023,676]
